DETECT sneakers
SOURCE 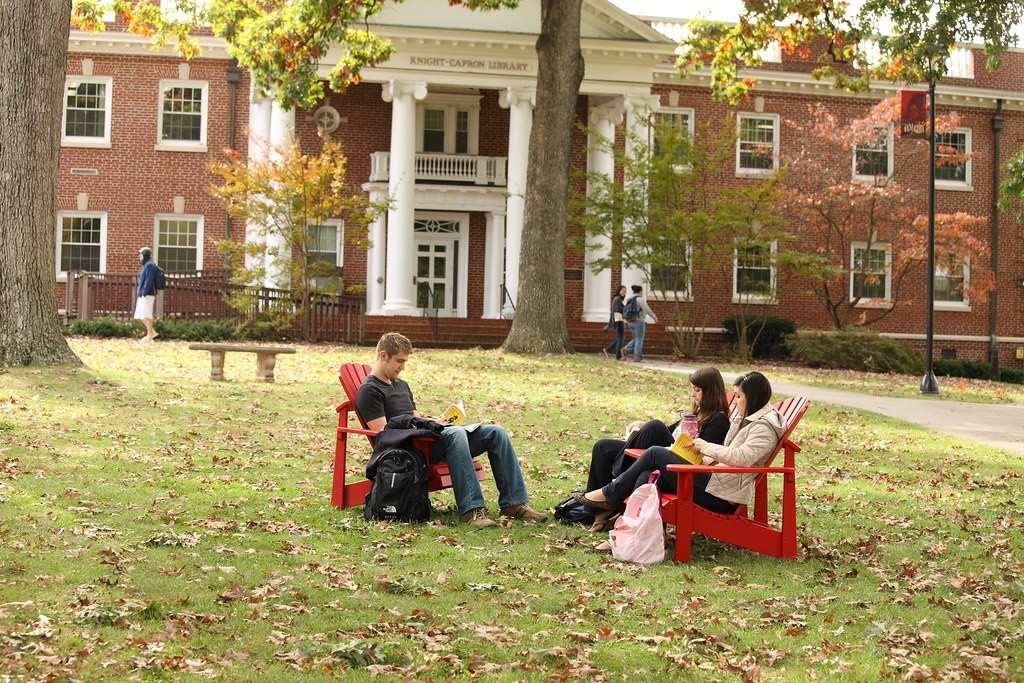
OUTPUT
[461,508,497,530]
[499,503,548,523]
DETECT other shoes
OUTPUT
[603,348,612,359]
[571,493,616,511]
[633,359,646,362]
[138,333,159,343]
[621,348,628,361]
[584,546,612,555]
[616,358,625,361]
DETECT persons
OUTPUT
[603,286,627,361]
[583,366,731,533]
[356,333,548,527]
[573,371,787,512]
[622,285,658,363]
[134,247,158,345]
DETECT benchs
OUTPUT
[188,343,296,383]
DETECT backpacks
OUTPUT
[146,262,165,289]
[623,296,642,323]
[609,470,668,564]
[554,491,591,523]
[364,447,431,523]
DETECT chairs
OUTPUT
[330,362,485,511]
[660,397,812,564]
[622,391,739,504]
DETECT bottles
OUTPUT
[680,412,699,438]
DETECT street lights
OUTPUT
[917,42,943,395]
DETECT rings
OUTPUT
[692,442,694,445]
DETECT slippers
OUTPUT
[591,512,620,532]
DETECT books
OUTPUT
[444,401,466,424]
[664,433,704,465]
